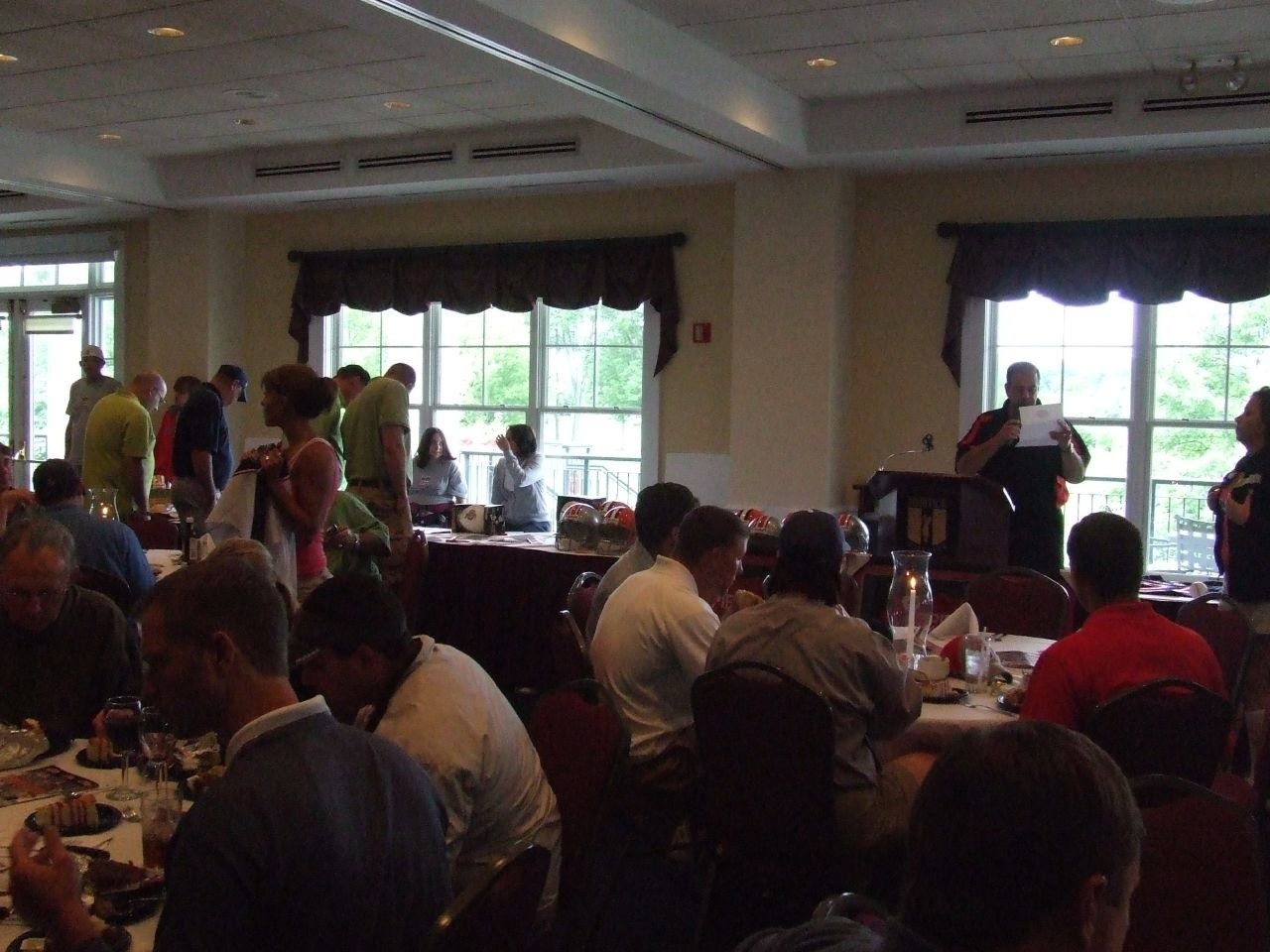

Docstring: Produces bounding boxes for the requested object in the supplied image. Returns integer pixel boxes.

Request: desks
[0,501,1226,952]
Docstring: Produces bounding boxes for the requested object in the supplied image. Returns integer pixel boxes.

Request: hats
[82,346,104,361]
[219,365,249,403]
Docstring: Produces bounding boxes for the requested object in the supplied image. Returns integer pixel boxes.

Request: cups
[963,633,999,707]
[140,787,184,879]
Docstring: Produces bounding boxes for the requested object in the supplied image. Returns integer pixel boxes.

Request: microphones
[1007,414,1022,448]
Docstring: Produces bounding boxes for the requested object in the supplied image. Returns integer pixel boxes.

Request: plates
[24,803,122,837]
[76,747,148,770]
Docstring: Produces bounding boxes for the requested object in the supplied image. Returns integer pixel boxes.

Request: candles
[102,506,109,519]
[907,576,917,659]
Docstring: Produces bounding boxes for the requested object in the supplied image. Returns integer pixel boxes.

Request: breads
[1003,688,1026,707]
[734,589,760,609]
[86,737,109,760]
[21,718,43,738]
[917,678,948,697]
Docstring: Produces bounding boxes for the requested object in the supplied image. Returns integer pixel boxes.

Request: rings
[276,452,281,457]
[265,455,270,461]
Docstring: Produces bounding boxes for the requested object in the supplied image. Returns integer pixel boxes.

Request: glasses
[0,578,69,606]
[153,388,167,405]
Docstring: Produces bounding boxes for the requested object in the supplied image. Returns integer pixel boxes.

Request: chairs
[1121,773,1270,952]
[548,608,697,952]
[526,675,630,924]
[566,569,602,633]
[1175,592,1256,714]
[692,661,841,951]
[418,844,551,952]
[965,567,1069,640]
[1089,679,1231,808]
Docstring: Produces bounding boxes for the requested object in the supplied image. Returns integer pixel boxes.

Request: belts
[350,478,395,488]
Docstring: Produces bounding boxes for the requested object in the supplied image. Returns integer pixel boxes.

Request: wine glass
[139,704,181,815]
[101,695,145,801]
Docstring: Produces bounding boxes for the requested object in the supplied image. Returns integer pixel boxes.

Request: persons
[241,363,343,609]
[93,539,297,750]
[340,364,420,636]
[705,507,940,860]
[739,717,1148,952]
[1020,511,1230,737]
[409,427,466,504]
[281,364,371,467]
[154,364,248,552]
[587,502,766,799]
[955,362,1091,579]
[491,424,551,533]
[1207,385,1270,792]
[0,343,166,743]
[584,481,701,650]
[322,491,391,581]
[8,555,450,952]
[291,572,562,952]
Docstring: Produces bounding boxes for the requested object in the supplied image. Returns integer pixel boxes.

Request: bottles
[182,516,200,563]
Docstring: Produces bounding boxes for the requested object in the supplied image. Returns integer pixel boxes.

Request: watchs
[1219,496,1231,511]
[1061,442,1074,453]
[354,532,360,548]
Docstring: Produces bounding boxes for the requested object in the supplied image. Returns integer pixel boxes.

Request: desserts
[34,792,99,827]
